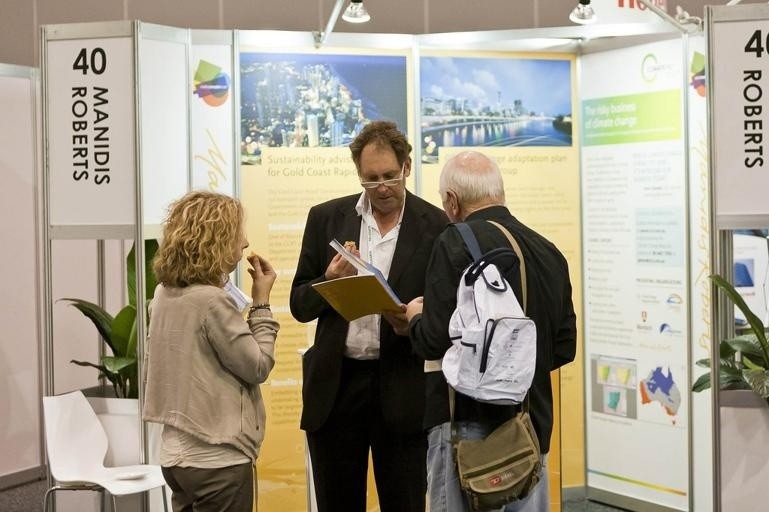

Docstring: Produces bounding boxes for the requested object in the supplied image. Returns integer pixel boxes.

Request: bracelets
[246,303,270,318]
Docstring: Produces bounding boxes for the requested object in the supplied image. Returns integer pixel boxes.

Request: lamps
[312,0,371,51]
[568,0,688,36]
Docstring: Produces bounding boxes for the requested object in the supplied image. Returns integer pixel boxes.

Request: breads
[344,241,355,248]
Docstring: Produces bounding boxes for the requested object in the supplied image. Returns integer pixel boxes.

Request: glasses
[361,160,405,189]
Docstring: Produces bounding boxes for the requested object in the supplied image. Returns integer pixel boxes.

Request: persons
[404,148,579,512]
[137,189,282,512]
[285,118,454,511]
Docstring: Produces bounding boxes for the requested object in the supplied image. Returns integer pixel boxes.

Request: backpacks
[441,222,537,406]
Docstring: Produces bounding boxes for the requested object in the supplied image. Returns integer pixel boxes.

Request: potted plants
[49,240,172,511]
[693,272,769,512]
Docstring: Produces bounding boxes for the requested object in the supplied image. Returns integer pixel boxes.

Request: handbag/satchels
[452,409,542,510]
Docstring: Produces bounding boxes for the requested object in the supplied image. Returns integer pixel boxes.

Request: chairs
[42,389,172,512]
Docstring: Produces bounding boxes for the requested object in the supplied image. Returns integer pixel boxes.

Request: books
[225,282,253,314]
[311,236,407,324]
